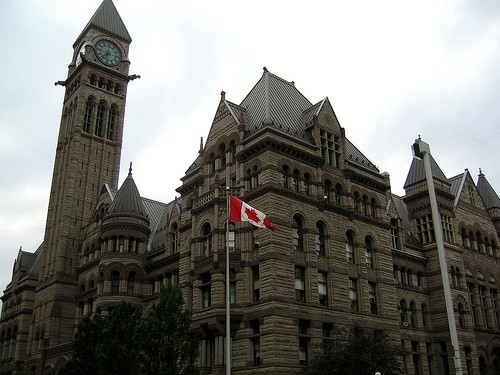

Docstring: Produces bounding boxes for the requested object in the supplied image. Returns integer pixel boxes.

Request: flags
[229,194,278,231]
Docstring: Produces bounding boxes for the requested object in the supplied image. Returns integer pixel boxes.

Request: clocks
[61,0,135,80]
